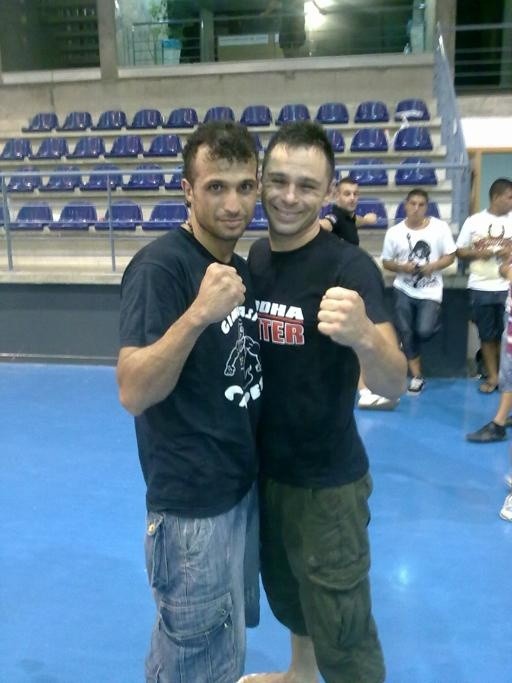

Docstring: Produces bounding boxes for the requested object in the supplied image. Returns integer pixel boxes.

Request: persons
[466,256,512,442]
[380,189,457,396]
[112,120,266,683]
[240,121,411,682]
[457,177,512,395]
[319,176,401,410]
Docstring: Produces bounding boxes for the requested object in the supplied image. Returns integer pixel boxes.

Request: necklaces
[414,218,426,228]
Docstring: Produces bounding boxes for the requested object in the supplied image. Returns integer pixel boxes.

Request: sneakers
[467,421,505,442]
[359,394,391,410]
[408,375,424,395]
[500,494,512,520]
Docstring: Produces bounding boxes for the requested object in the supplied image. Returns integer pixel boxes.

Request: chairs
[0,100,443,231]
